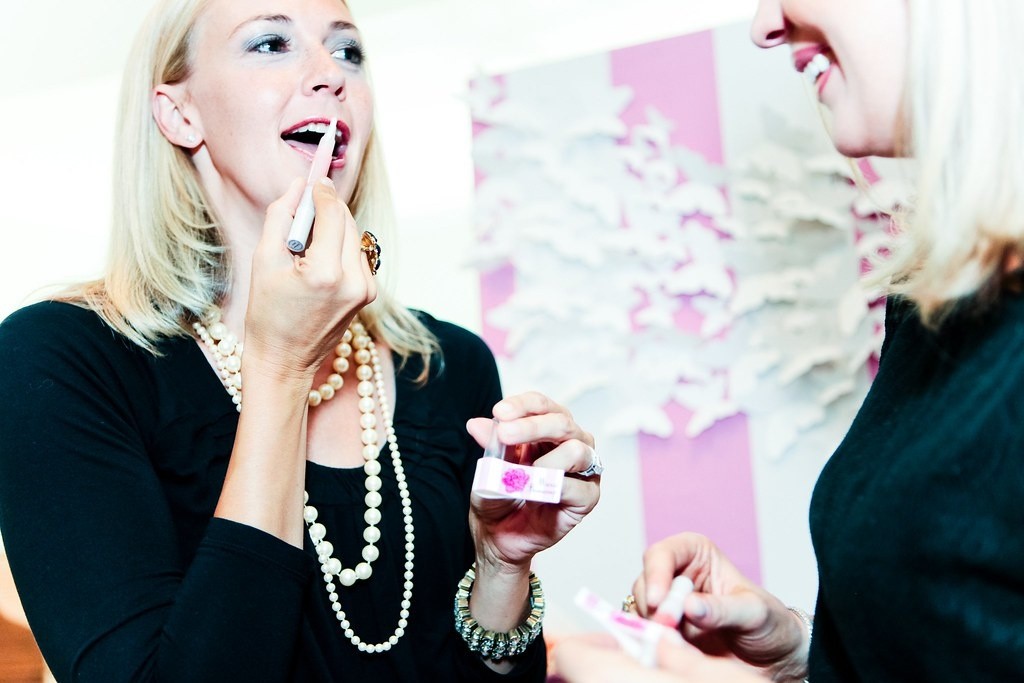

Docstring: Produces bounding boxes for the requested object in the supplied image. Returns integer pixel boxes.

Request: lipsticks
[287,118,337,252]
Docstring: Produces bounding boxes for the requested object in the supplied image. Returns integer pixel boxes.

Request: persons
[0,0,600,683]
[557,0,1024,683]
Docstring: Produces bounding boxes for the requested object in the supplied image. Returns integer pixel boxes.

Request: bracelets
[786,606,813,638]
[454,563,544,659]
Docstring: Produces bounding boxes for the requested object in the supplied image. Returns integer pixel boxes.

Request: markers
[286,113,340,253]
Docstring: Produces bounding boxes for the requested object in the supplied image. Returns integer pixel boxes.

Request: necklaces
[196,305,415,652]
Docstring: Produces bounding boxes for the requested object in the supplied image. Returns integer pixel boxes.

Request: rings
[360,230,381,274]
[578,447,604,477]
[622,596,637,612]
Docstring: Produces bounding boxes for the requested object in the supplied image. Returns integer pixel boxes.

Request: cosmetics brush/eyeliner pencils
[649,575,695,629]
